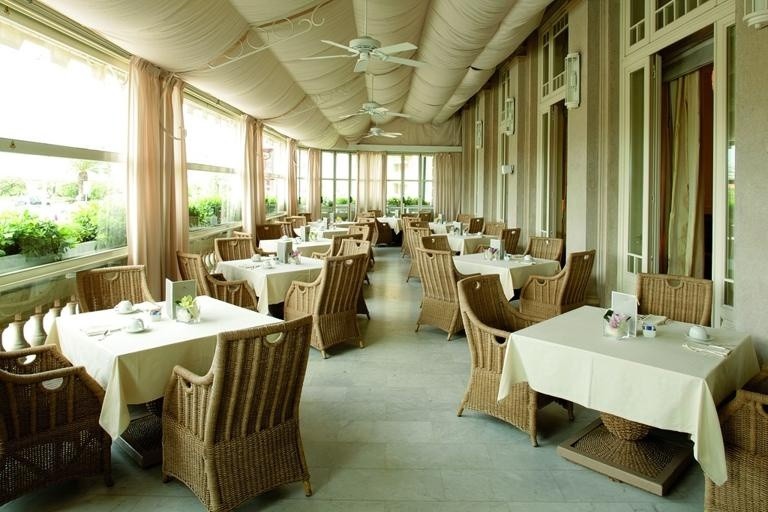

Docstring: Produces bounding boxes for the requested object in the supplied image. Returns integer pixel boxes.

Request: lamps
[564,51,582,110]
[743,0,767,31]
[475,120,483,149]
[502,98,514,134]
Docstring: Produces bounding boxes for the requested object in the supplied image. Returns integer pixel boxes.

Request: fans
[346,118,402,143]
[303,1,432,74]
[338,75,414,122]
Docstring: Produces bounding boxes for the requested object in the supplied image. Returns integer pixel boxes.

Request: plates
[117,310,135,313]
[127,329,145,333]
[686,332,714,341]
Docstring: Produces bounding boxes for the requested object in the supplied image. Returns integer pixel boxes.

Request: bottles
[150,311,161,321]
[642,325,656,337]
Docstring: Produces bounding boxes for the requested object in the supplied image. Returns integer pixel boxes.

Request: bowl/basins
[119,301,132,311]
[525,255,532,260]
[252,254,260,261]
[129,318,144,330]
[689,325,707,339]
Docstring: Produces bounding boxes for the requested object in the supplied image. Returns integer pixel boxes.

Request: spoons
[98,329,111,341]
